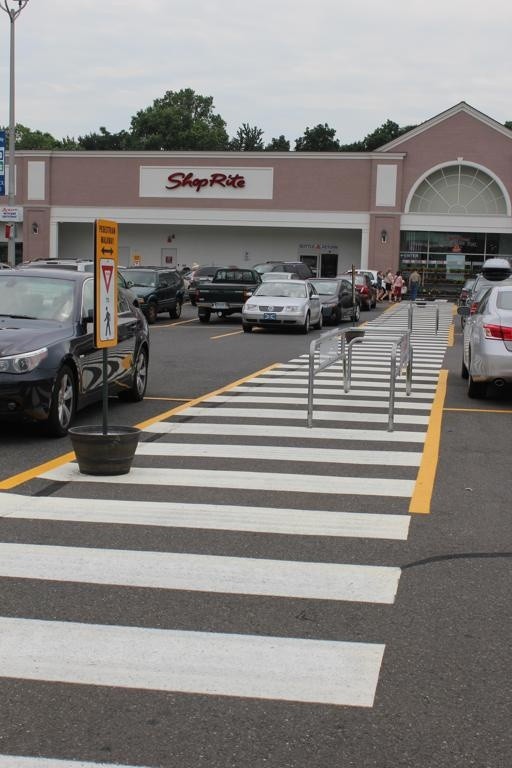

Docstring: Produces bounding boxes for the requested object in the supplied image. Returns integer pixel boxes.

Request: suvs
[119,265,184,323]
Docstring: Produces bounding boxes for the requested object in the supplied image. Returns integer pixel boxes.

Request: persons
[379,268,394,302]
[393,270,404,302]
[375,269,384,303]
[190,260,200,283]
[408,268,421,301]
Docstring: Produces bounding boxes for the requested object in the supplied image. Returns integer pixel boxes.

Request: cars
[457,258,512,399]
[0,263,149,437]
[177,262,384,334]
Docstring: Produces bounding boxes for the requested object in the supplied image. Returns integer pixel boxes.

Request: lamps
[381,229,387,244]
[32,222,39,235]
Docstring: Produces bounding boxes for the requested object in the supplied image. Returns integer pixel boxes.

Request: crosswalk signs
[94,220,119,349]
